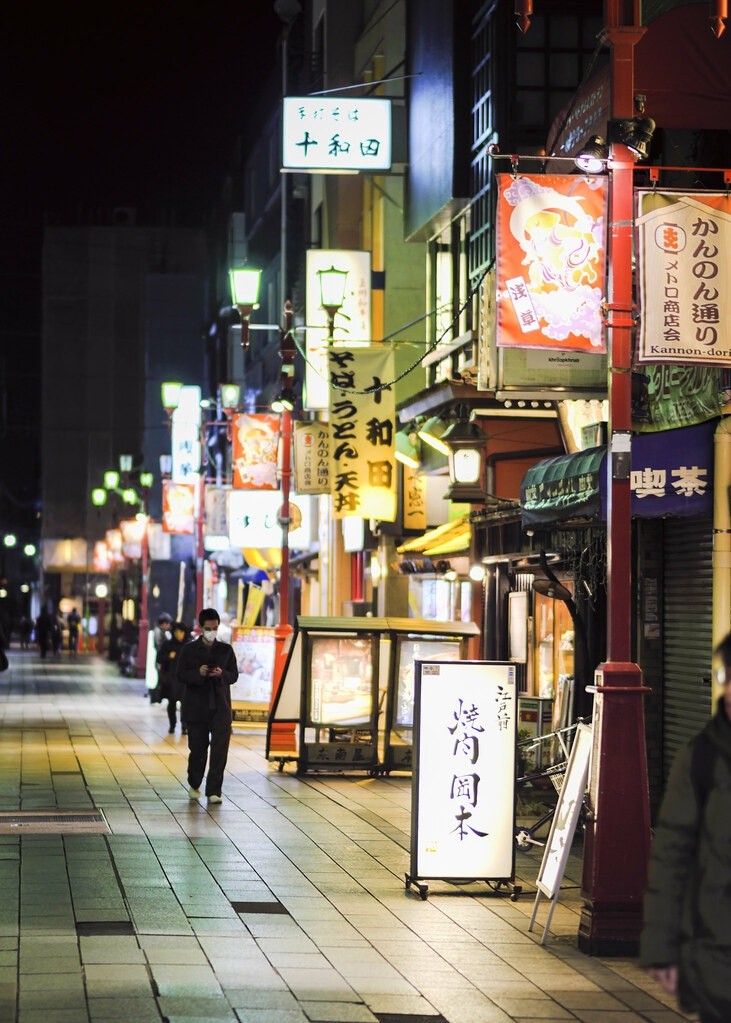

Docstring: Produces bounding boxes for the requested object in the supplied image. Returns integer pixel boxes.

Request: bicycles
[440,714,594,886]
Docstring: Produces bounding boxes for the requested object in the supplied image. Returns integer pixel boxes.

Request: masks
[202,628,218,641]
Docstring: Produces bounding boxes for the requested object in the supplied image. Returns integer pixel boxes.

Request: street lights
[86,378,242,701]
[228,254,348,750]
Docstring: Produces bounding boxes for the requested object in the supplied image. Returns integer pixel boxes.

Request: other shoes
[169,727,174,732]
[182,729,188,734]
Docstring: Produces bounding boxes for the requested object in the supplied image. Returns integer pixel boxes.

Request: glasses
[715,666,731,685]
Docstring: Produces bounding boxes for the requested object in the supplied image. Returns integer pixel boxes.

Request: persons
[149,613,178,708]
[176,607,240,804]
[215,614,233,645]
[155,623,193,737]
[643,634,731,1023]
[18,605,85,658]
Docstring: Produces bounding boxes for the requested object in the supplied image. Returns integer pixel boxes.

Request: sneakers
[208,795,221,804]
[188,786,201,800]
